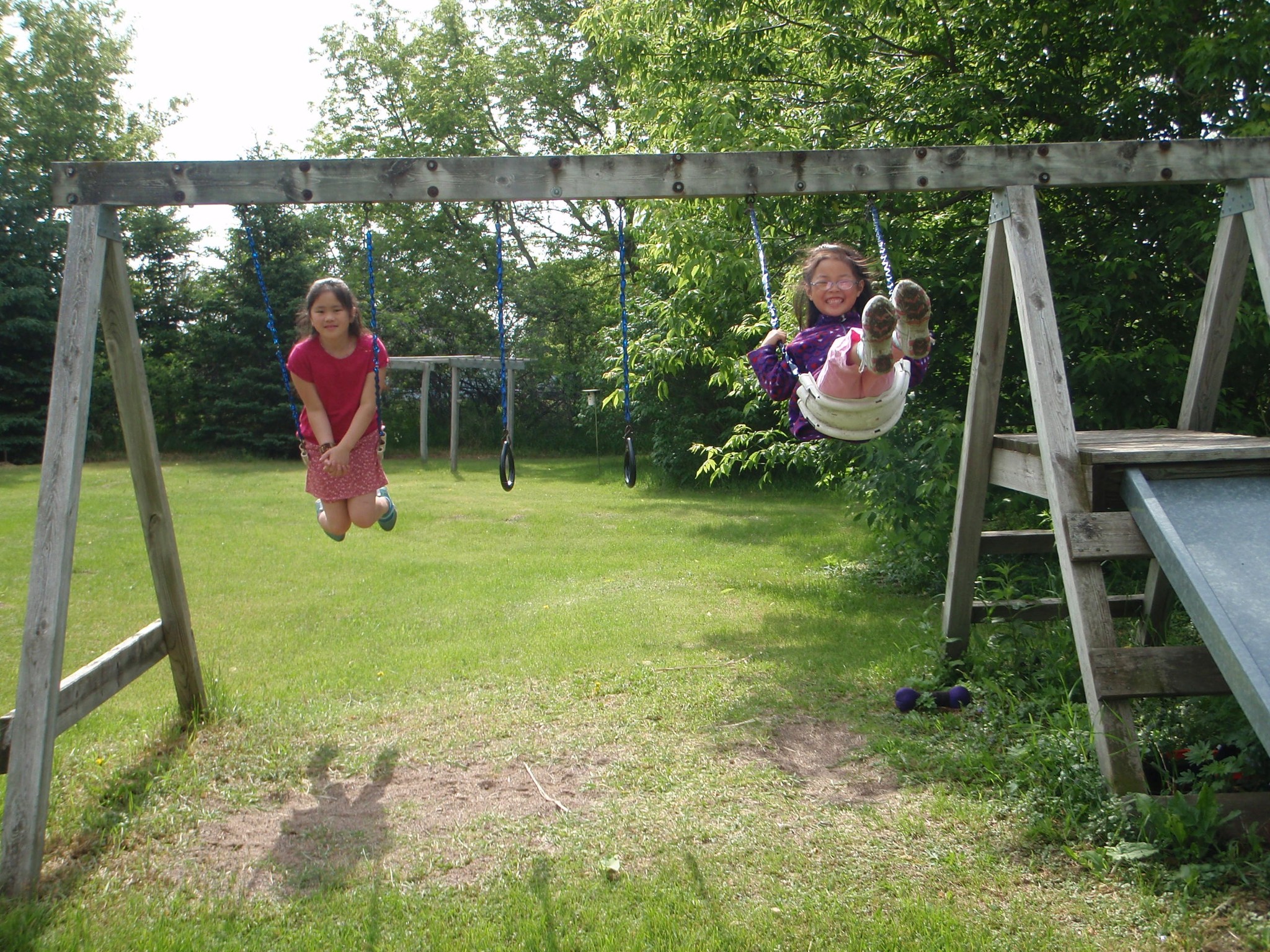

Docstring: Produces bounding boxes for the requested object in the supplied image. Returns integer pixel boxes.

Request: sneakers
[859,294,898,375]
[890,279,931,359]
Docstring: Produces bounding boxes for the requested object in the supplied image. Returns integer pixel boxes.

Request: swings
[744,190,912,441]
[236,201,389,469]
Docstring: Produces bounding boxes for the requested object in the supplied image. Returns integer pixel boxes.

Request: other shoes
[315,497,346,542]
[374,486,398,531]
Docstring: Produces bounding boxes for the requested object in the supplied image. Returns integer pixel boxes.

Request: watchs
[318,442,338,455]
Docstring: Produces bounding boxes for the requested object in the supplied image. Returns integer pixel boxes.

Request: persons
[287,275,397,540]
[746,243,930,444]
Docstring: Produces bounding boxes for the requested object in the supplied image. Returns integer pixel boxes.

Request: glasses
[809,280,859,291]
